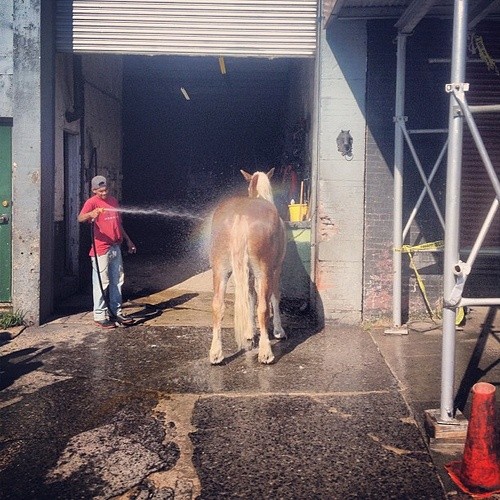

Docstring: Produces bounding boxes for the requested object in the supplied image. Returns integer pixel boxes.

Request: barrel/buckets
[288,204,307,222]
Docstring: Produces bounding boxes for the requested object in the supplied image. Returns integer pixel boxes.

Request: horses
[208,167,287,366]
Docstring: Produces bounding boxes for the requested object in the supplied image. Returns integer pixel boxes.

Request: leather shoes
[95,318,116,328]
[111,314,133,324]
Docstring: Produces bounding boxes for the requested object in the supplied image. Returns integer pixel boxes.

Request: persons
[78,175,136,329]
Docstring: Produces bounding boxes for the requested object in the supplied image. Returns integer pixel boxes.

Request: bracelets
[88,212,92,219]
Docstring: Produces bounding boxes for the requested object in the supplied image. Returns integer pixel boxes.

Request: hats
[91,175,107,190]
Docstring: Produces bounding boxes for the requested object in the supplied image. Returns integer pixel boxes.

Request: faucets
[96,207,105,213]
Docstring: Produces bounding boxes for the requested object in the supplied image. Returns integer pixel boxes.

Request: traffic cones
[443,382,500,495]
